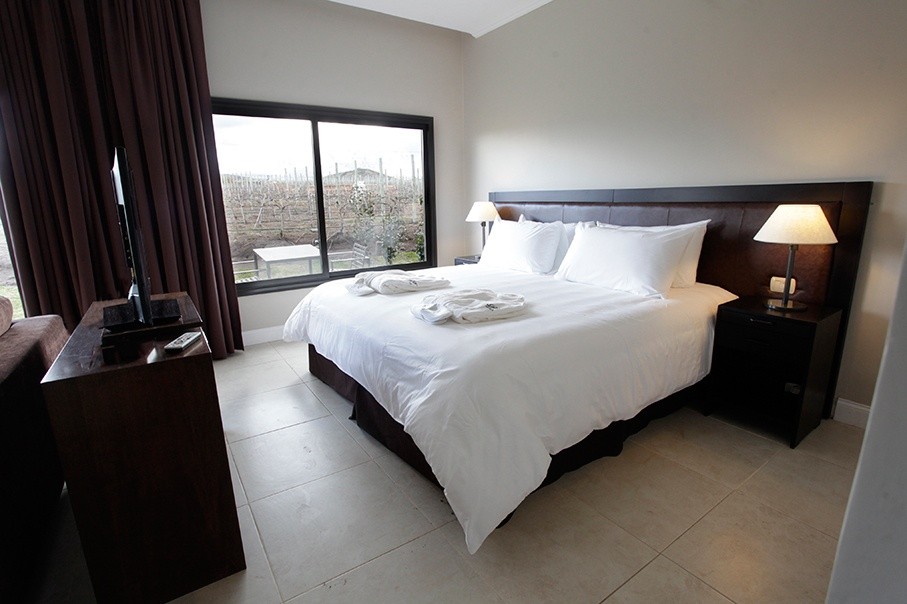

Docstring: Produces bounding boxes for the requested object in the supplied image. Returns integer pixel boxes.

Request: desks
[38,291,248,604]
[252,244,320,279]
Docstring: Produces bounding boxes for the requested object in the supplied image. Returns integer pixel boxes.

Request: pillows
[474,212,712,301]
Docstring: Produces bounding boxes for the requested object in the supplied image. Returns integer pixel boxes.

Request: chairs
[328,242,370,272]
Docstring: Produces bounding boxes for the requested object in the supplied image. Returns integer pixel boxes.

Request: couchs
[0,295,71,604]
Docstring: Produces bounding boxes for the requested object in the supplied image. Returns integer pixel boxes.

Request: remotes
[164,331,201,354]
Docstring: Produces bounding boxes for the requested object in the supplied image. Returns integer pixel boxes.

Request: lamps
[751,204,839,315]
[465,201,502,262]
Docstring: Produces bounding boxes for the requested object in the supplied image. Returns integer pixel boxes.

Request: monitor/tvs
[98,145,182,331]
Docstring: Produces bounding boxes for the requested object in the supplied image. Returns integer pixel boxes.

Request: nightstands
[707,290,853,450]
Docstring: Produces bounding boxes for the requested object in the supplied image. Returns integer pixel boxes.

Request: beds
[278,180,875,550]
